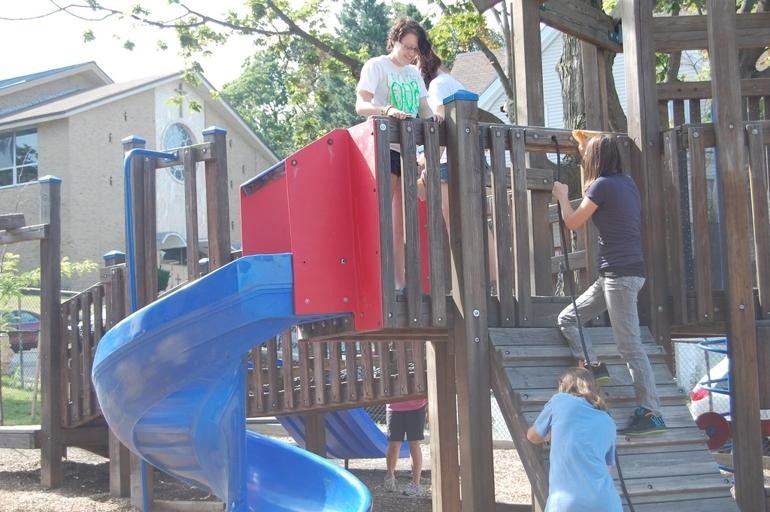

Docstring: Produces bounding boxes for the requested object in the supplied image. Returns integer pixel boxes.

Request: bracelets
[384,104,394,116]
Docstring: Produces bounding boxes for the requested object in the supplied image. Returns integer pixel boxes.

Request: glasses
[398,39,422,55]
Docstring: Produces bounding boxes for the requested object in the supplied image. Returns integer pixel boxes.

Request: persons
[552,129,667,438]
[526,366,623,512]
[411,46,500,299]
[353,19,445,305]
[385,398,428,498]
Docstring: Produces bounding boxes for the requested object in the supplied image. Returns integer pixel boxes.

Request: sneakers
[583,361,610,385]
[623,405,668,438]
[402,480,422,497]
[383,472,397,492]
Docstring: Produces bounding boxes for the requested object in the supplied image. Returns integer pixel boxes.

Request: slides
[91,253,378,511]
[246,360,410,469]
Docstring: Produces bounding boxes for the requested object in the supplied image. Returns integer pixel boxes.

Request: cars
[0,309,81,353]
[687,354,731,424]
